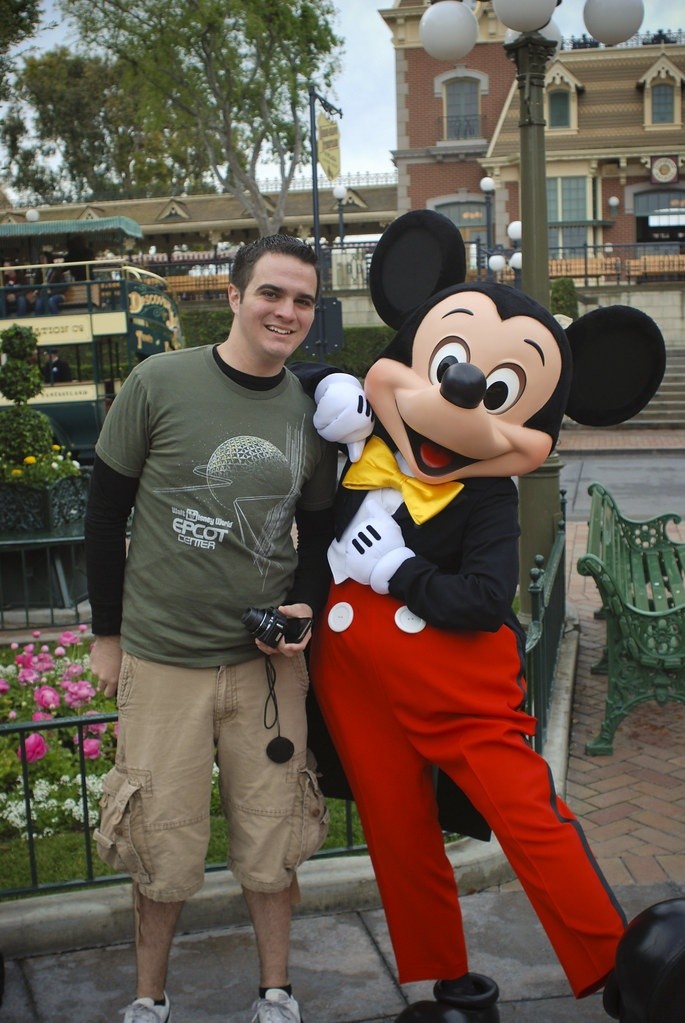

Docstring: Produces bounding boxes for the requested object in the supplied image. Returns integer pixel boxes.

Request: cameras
[240,606,290,648]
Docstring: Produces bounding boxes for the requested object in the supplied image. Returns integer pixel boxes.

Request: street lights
[332,184,347,254]
[479,175,522,291]
[420,0,644,646]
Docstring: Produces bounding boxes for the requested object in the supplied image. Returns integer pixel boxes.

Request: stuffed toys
[288,209,685,1023]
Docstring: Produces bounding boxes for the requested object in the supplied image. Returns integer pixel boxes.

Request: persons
[85,233,338,1023]
[31,251,68,317]
[0,260,28,317]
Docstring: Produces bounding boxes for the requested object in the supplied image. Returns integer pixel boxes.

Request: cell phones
[282,617,313,644]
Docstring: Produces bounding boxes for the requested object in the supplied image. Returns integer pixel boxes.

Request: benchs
[577,482,685,757]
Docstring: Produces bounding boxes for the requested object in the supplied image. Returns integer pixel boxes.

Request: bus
[0,217,186,456]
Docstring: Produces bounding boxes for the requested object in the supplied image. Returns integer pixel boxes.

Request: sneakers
[117,989,170,1023]
[250,988,301,1023]
